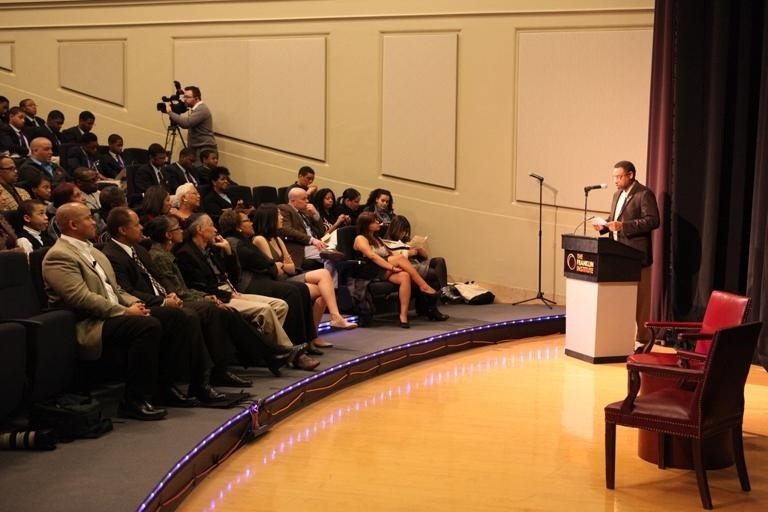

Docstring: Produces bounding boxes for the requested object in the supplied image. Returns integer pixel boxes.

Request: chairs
[605,289,764,510]
[0,139,414,441]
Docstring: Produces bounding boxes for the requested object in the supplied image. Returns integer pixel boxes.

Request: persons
[593,160,661,351]
[44,134,200,420]
[103,209,334,401]
[165,88,218,167]
[1,95,96,265]
[336,188,450,329]
[203,150,357,345]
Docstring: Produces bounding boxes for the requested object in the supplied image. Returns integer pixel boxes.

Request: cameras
[343,215,349,221]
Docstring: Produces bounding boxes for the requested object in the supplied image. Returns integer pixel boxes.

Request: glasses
[0,166,18,172]
[464,281,474,284]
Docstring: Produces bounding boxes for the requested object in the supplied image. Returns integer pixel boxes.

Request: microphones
[563,216,596,236]
[584,184,607,193]
[528,171,543,181]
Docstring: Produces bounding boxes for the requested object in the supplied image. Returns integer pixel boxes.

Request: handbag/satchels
[383,238,410,260]
[454,282,495,304]
[30,392,113,440]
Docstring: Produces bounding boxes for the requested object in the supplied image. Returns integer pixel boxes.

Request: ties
[131,246,167,298]
[116,153,124,169]
[186,169,194,186]
[158,171,163,184]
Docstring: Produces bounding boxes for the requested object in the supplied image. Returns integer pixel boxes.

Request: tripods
[163,125,187,167]
[512,180,557,309]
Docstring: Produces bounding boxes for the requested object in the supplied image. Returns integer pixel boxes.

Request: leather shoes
[439,286,465,305]
[264,340,334,376]
[117,368,252,421]
[398,314,410,328]
[426,307,450,321]
[330,320,358,330]
[19,131,29,156]
